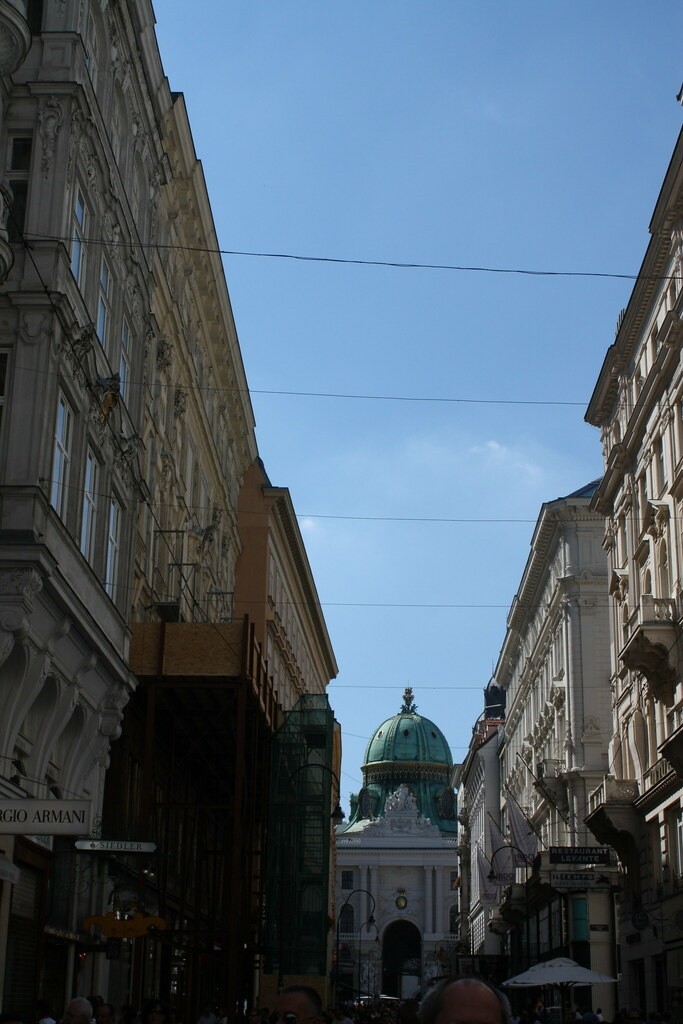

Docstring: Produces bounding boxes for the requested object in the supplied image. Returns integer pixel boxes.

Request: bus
[354,995,400,1007]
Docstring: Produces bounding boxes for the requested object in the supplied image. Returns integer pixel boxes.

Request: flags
[477,847,500,906]
[507,791,538,868]
[489,816,515,885]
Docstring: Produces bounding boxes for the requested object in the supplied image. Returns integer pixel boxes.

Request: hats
[383,1008,392,1016]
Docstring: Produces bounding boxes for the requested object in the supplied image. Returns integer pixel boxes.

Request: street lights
[482,844,530,969]
[334,889,379,1006]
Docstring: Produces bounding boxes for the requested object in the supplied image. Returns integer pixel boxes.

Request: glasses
[269,1012,316,1024]
[628,1017,642,1021]
[382,1015,388,1017]
[149,1010,162,1015]
[246,1014,257,1017]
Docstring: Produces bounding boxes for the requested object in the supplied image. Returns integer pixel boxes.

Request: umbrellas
[503,957,619,1024]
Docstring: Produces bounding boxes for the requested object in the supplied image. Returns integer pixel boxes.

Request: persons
[0,993,683,1024]
[416,977,511,1024]
[276,986,322,1024]
